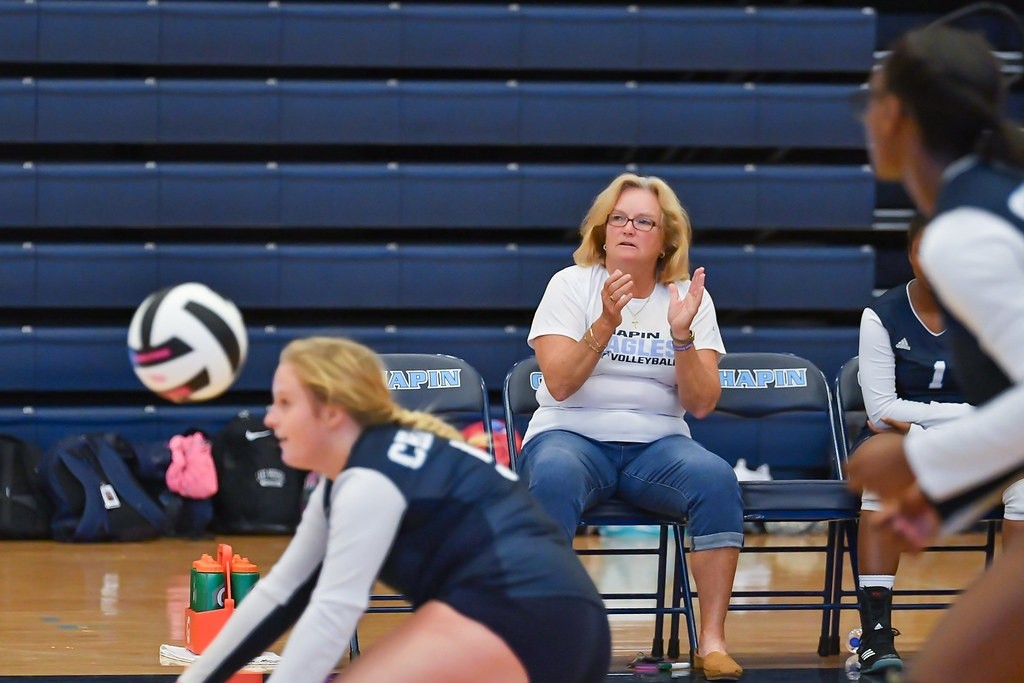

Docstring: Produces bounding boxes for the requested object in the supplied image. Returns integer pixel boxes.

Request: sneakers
[856,628,904,673]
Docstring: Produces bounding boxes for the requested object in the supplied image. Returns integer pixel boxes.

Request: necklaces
[622,280,657,329]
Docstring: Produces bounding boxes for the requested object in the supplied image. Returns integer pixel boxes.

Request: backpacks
[47,431,170,541]
[212,417,298,535]
[1,433,44,539]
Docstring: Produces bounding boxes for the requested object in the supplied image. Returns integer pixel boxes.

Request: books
[158,645,281,675]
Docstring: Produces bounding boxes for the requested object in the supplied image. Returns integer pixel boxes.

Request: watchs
[670,328,695,344]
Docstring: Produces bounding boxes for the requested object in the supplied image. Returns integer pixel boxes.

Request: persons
[512,173,745,682]
[174,336,610,683]
[844,27,1024,683]
[849,211,1024,673]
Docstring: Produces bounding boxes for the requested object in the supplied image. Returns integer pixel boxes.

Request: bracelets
[589,324,609,350]
[671,342,695,352]
[582,334,603,353]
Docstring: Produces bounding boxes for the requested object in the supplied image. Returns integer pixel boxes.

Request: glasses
[607,213,663,232]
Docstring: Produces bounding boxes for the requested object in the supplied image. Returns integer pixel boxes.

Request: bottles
[845,629,863,654]
[190,554,225,611]
[233,552,260,608]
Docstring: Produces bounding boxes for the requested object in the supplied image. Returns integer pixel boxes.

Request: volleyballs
[126,281,249,404]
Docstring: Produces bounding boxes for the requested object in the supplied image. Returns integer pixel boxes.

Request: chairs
[351,352,496,661]
[667,353,863,666]
[828,355,995,655]
[502,356,697,668]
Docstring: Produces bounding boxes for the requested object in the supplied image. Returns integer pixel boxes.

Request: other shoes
[693,649,742,681]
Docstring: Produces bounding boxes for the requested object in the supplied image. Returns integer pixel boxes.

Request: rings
[608,296,618,303]
